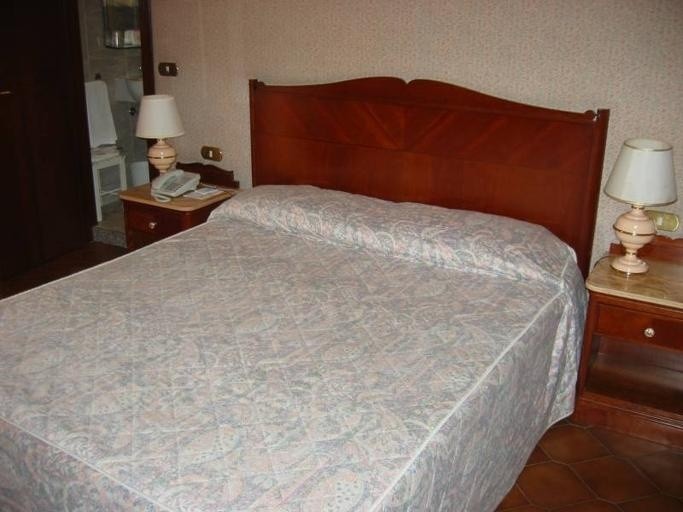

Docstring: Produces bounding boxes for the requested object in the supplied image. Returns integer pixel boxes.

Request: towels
[85,79,118,148]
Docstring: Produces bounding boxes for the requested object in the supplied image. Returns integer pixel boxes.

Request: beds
[0,77,611,512]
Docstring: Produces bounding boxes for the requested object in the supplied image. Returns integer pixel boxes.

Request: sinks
[116,75,143,102]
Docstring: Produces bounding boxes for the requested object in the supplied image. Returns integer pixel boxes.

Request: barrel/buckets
[130,161,150,186]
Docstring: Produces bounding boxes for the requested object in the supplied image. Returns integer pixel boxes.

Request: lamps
[603,136,678,279]
[134,93,186,176]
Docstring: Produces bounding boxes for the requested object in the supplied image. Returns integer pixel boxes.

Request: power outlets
[643,210,680,233]
[200,145,223,162]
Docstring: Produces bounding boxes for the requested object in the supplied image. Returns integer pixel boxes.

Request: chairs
[84,80,128,222]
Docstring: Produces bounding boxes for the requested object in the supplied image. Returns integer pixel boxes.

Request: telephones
[151,169,201,197]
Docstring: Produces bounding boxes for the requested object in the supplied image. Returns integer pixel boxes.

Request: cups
[109,31,139,46]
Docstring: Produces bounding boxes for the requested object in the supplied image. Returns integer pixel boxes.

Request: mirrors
[101,0,142,51]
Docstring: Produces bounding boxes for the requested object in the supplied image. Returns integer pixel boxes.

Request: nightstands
[568,235,683,450]
[117,162,241,254]
